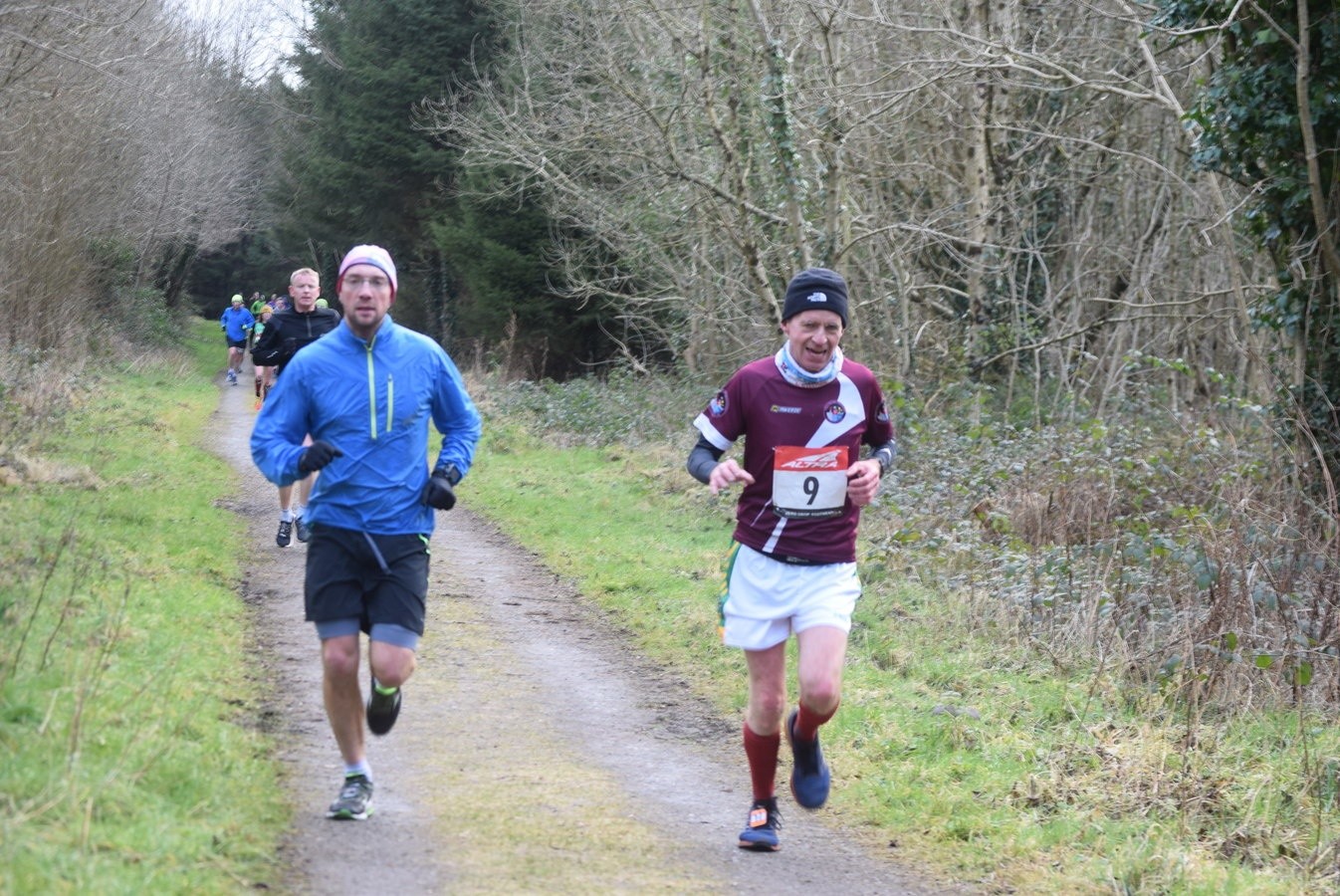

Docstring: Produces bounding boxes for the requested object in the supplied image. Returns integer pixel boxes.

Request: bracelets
[868,457,887,480]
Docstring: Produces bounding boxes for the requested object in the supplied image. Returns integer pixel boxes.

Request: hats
[316,299,328,310]
[782,267,848,330]
[260,305,272,314]
[337,244,399,305]
[231,295,243,302]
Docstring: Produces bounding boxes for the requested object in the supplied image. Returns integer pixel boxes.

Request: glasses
[342,275,389,292]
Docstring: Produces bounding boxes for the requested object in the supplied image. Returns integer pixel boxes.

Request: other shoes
[256,397,262,409]
[227,369,237,385]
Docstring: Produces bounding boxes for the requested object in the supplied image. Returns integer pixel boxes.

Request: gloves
[241,325,247,330]
[222,326,226,331]
[421,470,457,511]
[298,439,342,473]
[277,336,297,360]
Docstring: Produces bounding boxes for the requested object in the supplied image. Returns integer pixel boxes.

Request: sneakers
[366,676,401,735]
[784,711,830,809]
[740,797,784,851]
[324,771,374,820]
[294,515,312,543]
[277,514,294,547]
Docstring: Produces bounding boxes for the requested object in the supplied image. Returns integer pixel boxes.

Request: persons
[314,297,329,310]
[250,266,341,549]
[220,290,292,410]
[249,244,483,822]
[686,268,892,853]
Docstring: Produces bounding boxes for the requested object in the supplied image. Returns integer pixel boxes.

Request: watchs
[431,462,463,486]
